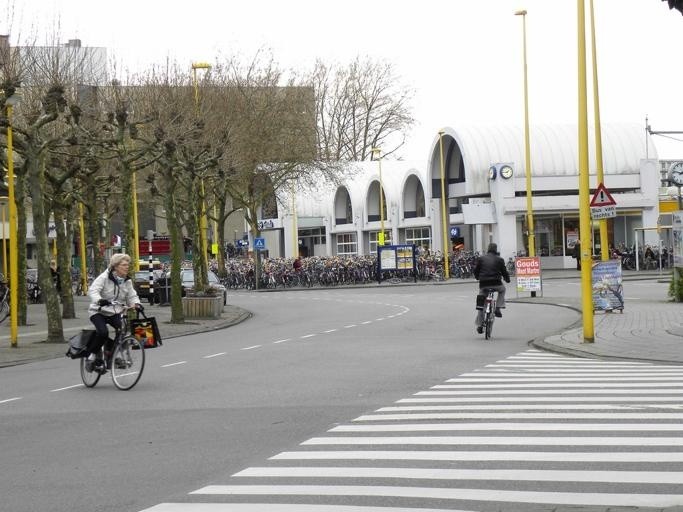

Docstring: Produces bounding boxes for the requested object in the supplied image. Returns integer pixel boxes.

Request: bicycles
[472,274,509,342]
[72,297,153,388]
[0,275,41,327]
[73,273,93,297]
[502,239,674,276]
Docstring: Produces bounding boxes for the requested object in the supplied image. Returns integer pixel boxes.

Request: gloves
[135,304,144,312]
[97,297,111,307]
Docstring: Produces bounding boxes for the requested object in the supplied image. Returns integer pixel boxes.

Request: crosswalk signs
[253,238,266,250]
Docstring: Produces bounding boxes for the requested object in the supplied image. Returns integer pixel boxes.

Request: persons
[473,243,510,334]
[572,239,580,271]
[83,253,141,371]
[415,245,422,255]
[422,244,430,259]
[293,255,302,272]
[48,258,55,273]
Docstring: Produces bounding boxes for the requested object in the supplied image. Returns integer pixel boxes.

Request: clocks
[487,166,497,180]
[498,164,514,179]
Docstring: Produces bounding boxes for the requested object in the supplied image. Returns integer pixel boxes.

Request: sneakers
[495,310,502,318]
[83,355,94,372]
[476,326,484,334]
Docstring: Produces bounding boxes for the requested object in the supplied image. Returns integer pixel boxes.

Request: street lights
[370,146,387,249]
[434,127,451,281]
[513,9,539,296]
[188,51,215,273]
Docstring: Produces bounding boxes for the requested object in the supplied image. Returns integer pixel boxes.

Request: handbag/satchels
[129,306,163,350]
[65,328,98,359]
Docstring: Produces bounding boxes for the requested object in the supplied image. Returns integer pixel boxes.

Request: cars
[124,256,164,304]
[23,264,81,297]
[161,268,229,307]
